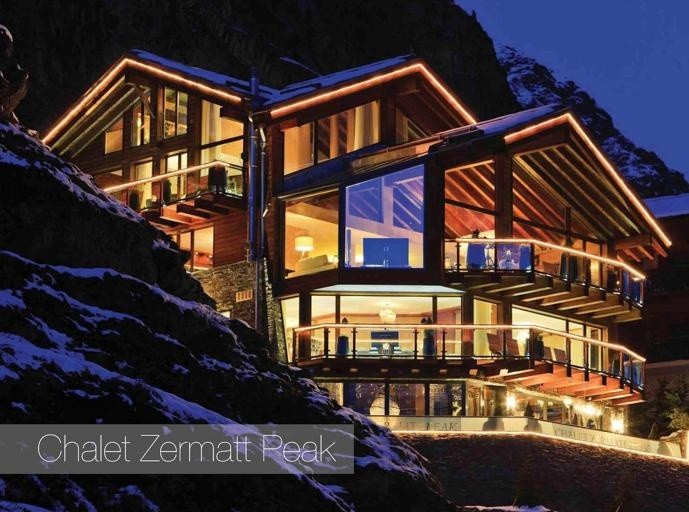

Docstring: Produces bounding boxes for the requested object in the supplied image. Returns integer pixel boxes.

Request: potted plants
[667,407,689,459]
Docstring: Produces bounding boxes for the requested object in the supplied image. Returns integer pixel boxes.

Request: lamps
[380,306,397,324]
[295,235,315,257]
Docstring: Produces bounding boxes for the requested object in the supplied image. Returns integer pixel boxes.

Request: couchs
[288,255,336,278]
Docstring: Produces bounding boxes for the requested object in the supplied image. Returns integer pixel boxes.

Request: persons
[606,264,617,291]
[537,335,544,359]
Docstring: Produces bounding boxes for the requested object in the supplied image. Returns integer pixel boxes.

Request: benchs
[488,333,525,360]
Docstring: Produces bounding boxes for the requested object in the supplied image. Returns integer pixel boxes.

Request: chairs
[543,346,552,361]
[554,348,566,363]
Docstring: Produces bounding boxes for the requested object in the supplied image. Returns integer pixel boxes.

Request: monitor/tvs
[363,237,409,265]
[370,331,402,351]
[495,244,534,271]
